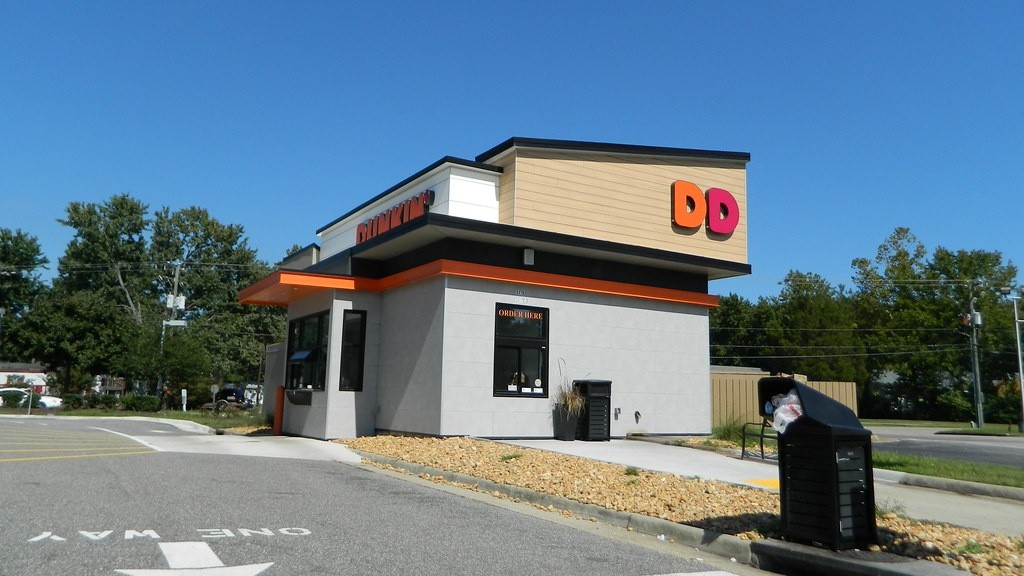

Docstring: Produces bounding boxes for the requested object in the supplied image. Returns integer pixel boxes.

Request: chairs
[741,418,775,459]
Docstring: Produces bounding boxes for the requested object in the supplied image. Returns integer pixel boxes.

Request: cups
[299,383,303,388]
[307,385,312,388]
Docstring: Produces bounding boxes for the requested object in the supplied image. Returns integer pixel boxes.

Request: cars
[0,387,64,410]
[214,382,265,414]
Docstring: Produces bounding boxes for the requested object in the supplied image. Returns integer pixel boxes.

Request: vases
[552,406,578,441]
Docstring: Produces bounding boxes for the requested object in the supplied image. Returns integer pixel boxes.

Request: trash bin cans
[757,375,878,552]
[571,379,612,442]
